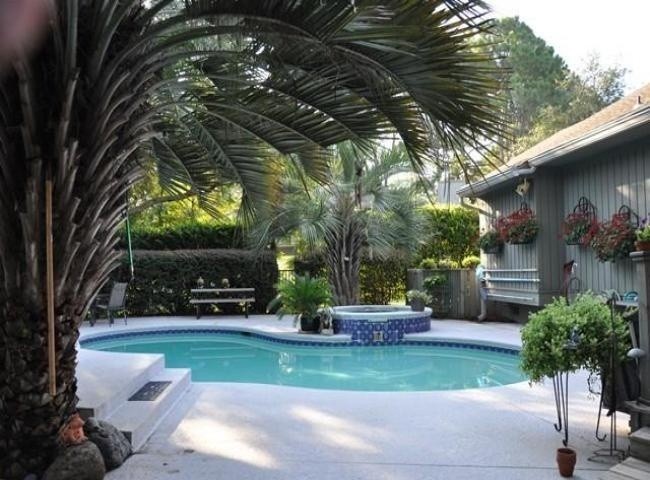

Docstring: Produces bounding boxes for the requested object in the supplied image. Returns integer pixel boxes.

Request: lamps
[513,180,530,202]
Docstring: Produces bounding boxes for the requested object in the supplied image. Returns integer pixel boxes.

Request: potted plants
[462,254,480,270]
[272,271,336,332]
[404,288,433,311]
[634,225,650,252]
[422,257,434,269]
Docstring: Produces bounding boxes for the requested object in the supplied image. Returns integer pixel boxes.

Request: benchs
[189,276,256,320]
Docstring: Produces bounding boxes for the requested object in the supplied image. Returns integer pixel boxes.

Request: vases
[556,449,577,478]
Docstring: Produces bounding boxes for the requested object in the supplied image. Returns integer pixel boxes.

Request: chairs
[90,282,128,327]
